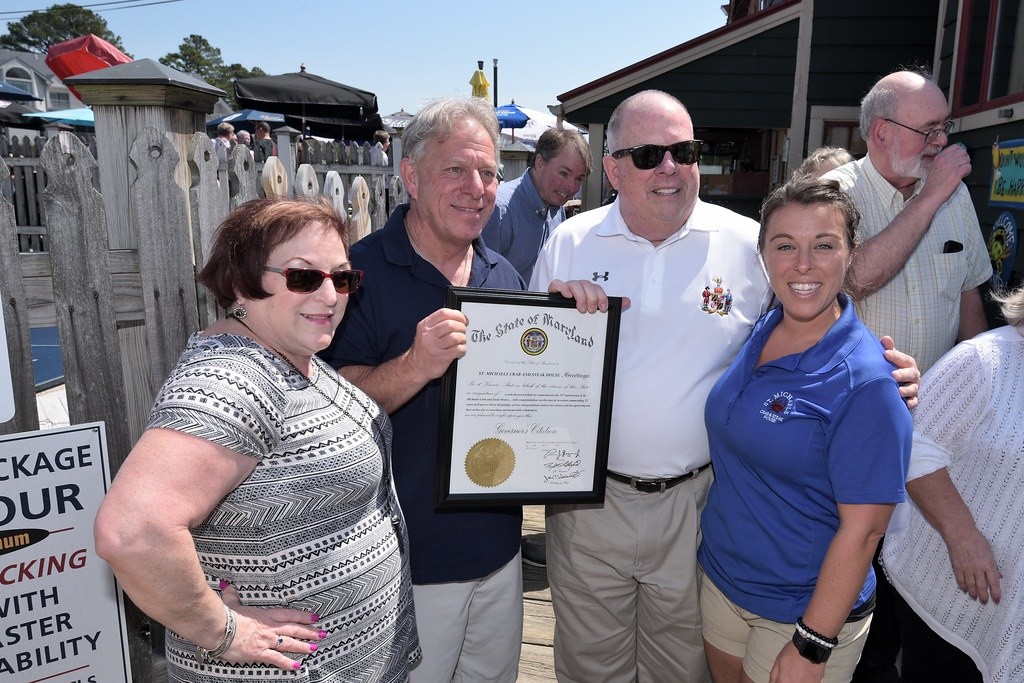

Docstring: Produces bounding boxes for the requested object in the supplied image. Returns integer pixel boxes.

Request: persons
[851,294,1024,683]
[697,178,912,683]
[372,129,390,214]
[528,90,920,683]
[94,198,423,683]
[317,97,529,683]
[819,72,993,374]
[212,124,302,184]
[482,129,592,284]
[801,147,854,178]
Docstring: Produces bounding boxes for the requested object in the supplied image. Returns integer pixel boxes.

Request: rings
[276,635,283,649]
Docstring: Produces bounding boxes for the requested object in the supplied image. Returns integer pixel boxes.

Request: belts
[607,463,712,494]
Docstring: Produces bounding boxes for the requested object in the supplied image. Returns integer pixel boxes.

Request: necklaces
[232,315,405,554]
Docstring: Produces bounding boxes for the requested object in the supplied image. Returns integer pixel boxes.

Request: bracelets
[796,616,838,648]
[195,605,236,664]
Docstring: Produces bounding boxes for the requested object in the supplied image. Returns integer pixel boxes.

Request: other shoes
[522,541,547,567]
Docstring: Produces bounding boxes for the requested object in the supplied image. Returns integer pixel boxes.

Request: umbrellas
[495,99,578,143]
[470,70,491,100]
[205,62,384,140]
[0,80,94,126]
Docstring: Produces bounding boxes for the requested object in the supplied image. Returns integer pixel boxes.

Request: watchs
[792,630,831,663]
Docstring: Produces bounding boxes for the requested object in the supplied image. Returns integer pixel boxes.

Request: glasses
[883,118,955,144]
[262,266,363,294]
[612,139,704,170]
